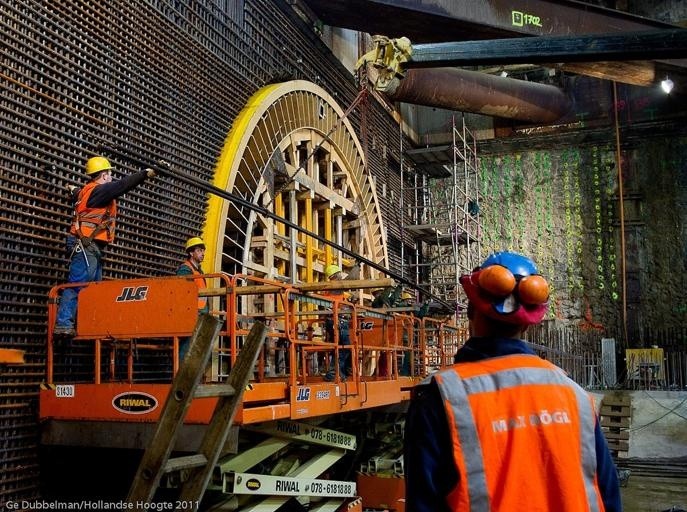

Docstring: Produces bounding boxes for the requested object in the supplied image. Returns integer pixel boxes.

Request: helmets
[327,265,341,277]
[85,156,115,175]
[402,293,413,299]
[461,253,549,325]
[185,238,206,252]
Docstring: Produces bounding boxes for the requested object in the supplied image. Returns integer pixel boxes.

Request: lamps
[660,74,675,94]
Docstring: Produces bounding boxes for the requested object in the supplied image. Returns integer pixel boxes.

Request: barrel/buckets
[616,467,630,488]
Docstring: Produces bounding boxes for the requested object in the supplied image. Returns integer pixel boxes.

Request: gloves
[66,184,79,194]
[146,159,170,177]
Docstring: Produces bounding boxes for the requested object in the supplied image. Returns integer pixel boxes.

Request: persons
[52,155,171,339]
[175,235,210,382]
[372,283,403,374]
[402,250,623,511]
[401,292,433,377]
[323,263,360,385]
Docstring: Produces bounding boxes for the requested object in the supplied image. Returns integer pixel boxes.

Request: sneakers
[55,327,74,334]
